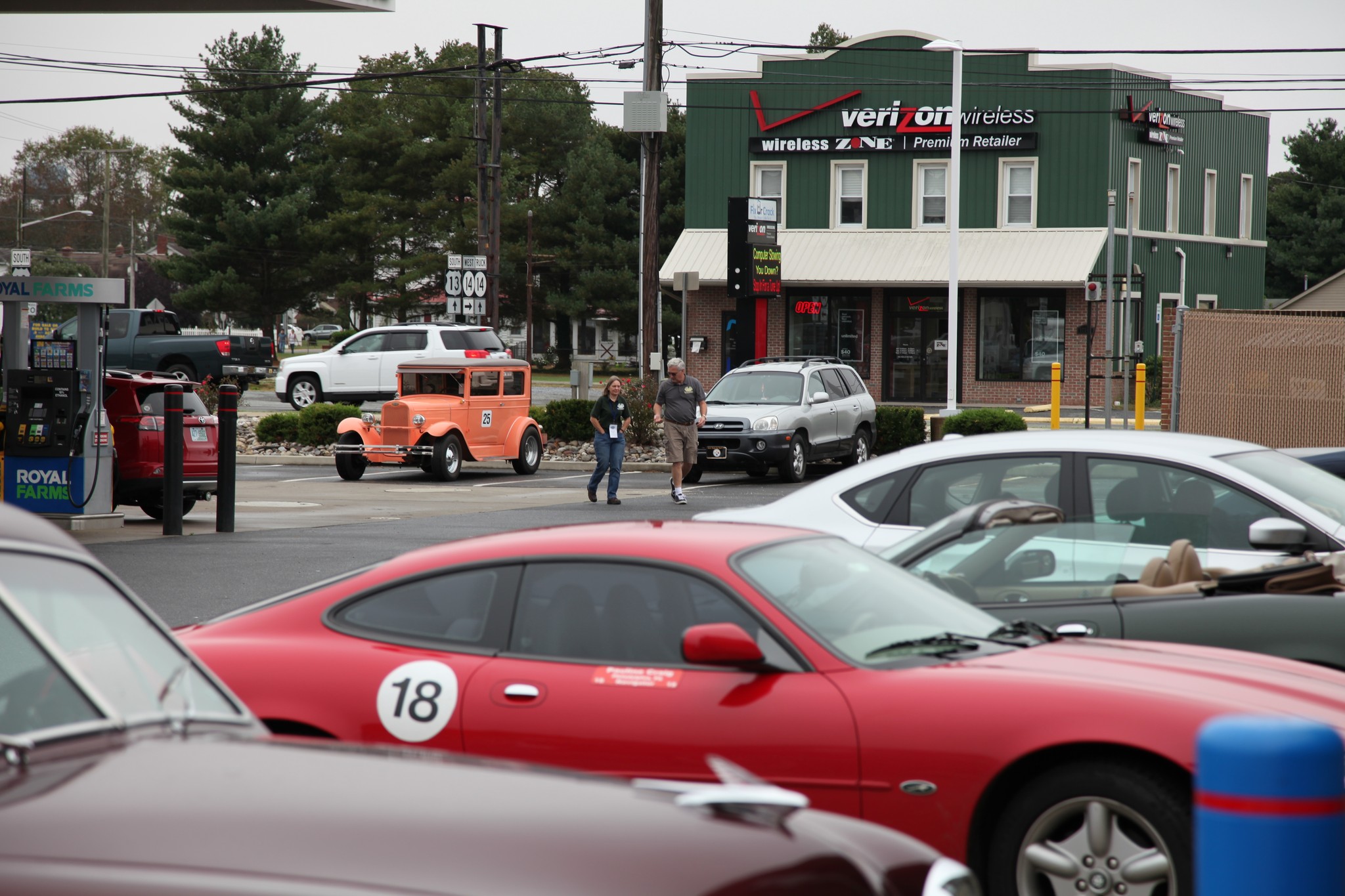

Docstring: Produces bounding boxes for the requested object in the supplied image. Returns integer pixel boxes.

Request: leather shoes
[607,497,621,504]
[587,484,598,502]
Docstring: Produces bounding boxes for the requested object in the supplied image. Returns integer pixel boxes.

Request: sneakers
[674,493,687,504]
[670,477,676,501]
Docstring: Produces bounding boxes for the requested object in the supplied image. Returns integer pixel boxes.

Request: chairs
[742,379,768,402]
[344,576,686,663]
[978,470,1234,549]
[413,376,517,395]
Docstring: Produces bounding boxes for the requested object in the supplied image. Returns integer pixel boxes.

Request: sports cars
[859,502,1344,671]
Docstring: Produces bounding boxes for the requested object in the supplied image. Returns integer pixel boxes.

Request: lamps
[1226,246,1233,257]
[1150,240,1157,252]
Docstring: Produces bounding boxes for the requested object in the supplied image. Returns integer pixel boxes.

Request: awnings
[658,229,1112,290]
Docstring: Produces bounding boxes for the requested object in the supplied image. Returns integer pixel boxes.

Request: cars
[273,323,305,347]
[334,354,543,482]
[1175,445,1345,512]
[1027,316,1065,381]
[3,519,1345,891]
[691,443,1345,604]
[0,506,985,896]
[891,328,948,360]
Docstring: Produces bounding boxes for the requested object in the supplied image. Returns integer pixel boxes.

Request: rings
[702,425,703,426]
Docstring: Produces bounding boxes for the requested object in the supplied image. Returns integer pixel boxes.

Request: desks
[892,361,948,400]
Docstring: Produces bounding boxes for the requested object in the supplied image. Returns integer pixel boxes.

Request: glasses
[666,369,681,377]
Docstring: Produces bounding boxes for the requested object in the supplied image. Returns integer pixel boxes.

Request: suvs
[273,321,518,411]
[301,324,346,344]
[684,356,880,482]
[102,368,218,522]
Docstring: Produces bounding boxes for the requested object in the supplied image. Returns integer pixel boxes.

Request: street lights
[14,190,94,252]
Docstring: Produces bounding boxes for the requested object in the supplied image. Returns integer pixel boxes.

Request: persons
[652,358,707,505]
[278,329,299,354]
[587,376,632,505]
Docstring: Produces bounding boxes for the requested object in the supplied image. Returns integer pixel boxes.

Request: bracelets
[702,414,707,420]
[620,429,624,433]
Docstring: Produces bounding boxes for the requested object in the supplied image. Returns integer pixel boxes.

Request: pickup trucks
[41,306,275,402]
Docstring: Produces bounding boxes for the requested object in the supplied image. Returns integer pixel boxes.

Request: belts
[664,417,695,426]
